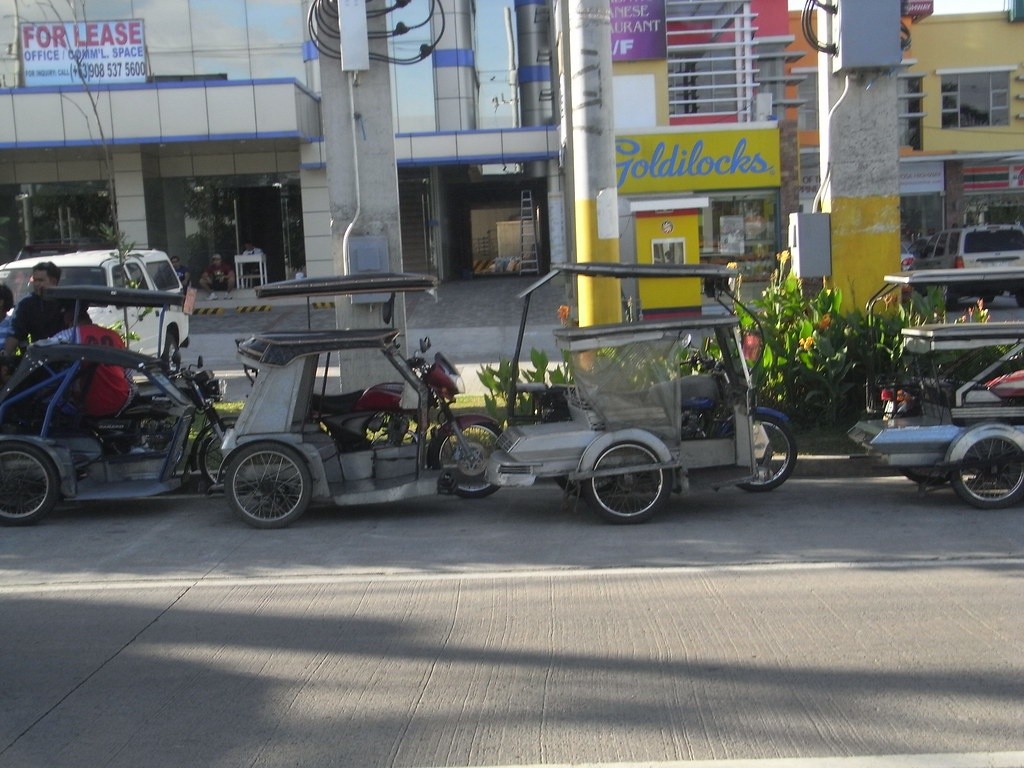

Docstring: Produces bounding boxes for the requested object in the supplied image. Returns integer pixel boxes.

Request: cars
[901,244,914,270]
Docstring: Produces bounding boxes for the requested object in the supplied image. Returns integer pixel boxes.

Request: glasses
[172,260,179,263]
[212,258,220,260]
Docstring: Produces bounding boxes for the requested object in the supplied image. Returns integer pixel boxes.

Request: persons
[25,304,145,457]
[241,240,264,272]
[0,283,19,386]
[170,255,192,294]
[12,261,75,355]
[199,253,237,302]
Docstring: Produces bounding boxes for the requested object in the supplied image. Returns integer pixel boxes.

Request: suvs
[924,224,1024,305]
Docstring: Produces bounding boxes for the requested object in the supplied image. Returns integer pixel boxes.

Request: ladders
[518,190,541,277]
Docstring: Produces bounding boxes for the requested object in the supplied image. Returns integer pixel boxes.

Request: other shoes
[224,293,233,300]
[206,292,218,300]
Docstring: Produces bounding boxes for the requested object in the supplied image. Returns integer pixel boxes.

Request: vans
[2,251,191,375]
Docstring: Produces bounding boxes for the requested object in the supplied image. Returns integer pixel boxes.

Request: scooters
[848,269,1024,511]
[0,283,243,523]
[215,271,506,528]
[482,261,800,523]
